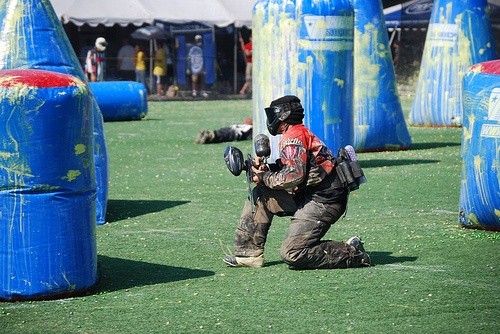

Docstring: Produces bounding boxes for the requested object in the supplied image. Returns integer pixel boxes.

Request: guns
[253,133,272,171]
[224,144,257,215]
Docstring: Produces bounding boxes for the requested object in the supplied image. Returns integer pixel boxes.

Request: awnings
[50,0,258,29]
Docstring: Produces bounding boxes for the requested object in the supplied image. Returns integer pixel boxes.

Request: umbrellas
[382,0,500,31]
[131,26,171,95]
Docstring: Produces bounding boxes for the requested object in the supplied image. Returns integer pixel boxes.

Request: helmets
[95,37,107,51]
[265,96,304,136]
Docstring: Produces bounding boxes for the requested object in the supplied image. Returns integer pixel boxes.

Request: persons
[117,37,135,81]
[131,45,147,86]
[239,36,252,95]
[223,95,372,268]
[196,119,253,145]
[85,37,108,82]
[152,40,167,96]
[187,34,209,98]
[393,41,411,74]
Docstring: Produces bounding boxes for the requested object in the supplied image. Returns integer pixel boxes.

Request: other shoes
[192,90,197,97]
[200,92,209,97]
[195,129,210,145]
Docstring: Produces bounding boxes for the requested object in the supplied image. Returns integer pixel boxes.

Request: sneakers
[347,237,371,267]
[222,254,265,269]
